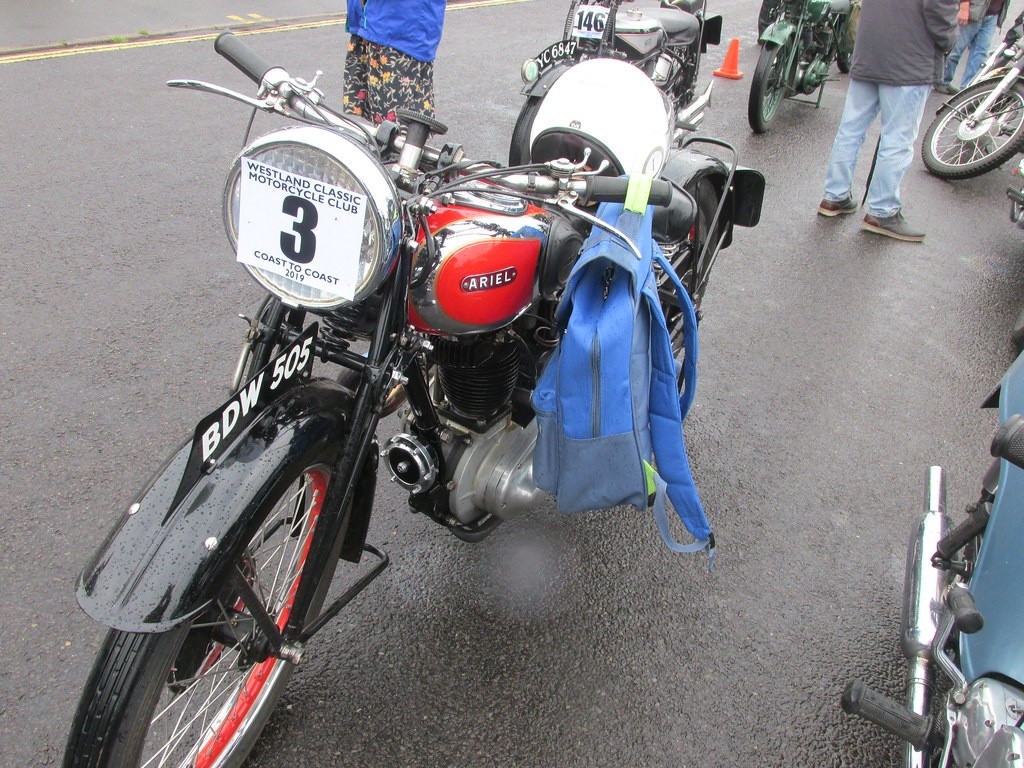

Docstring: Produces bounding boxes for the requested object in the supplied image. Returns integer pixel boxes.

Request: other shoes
[935,84,960,95]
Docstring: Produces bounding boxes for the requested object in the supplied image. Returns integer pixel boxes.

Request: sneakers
[818,190,858,217]
[861,207,925,241]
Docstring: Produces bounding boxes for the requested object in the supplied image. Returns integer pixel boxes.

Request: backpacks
[530,174,717,573]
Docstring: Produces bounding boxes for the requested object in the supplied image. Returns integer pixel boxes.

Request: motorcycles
[57,28,771,768]
[506,1,724,175]
[748,0,863,134]
[839,300,1024,768]
[920,9,1024,181]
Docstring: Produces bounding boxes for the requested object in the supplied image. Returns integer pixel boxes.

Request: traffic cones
[712,38,744,80]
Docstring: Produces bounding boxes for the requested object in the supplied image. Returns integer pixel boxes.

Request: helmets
[530,57,670,179]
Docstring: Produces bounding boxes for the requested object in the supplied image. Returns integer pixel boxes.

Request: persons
[938,0,1010,93]
[817,0,961,241]
[343,0,446,137]
